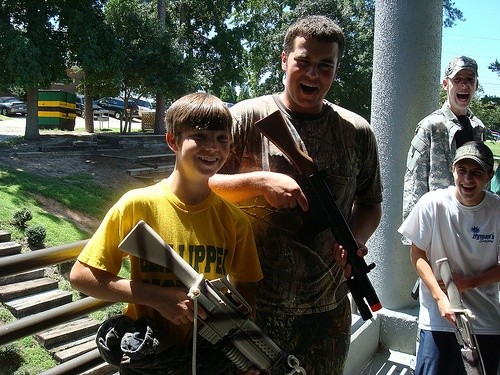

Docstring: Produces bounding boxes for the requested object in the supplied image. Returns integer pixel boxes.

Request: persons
[205,15,382,375]
[69,92,258,375]
[410,140,500,375]
[403,56,487,245]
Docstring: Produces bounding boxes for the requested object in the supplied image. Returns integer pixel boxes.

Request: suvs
[94,97,140,120]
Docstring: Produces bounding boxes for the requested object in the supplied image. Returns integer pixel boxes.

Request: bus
[132,96,172,119]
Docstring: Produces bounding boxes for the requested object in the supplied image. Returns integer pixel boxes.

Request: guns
[433,255,487,375]
[116,220,309,375]
[250,107,382,320]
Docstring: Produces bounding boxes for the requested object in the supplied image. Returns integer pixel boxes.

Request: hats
[452,141,494,171]
[444,56,479,79]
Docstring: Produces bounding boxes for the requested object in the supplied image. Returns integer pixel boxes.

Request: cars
[0,97,28,116]
[76,96,102,120]
